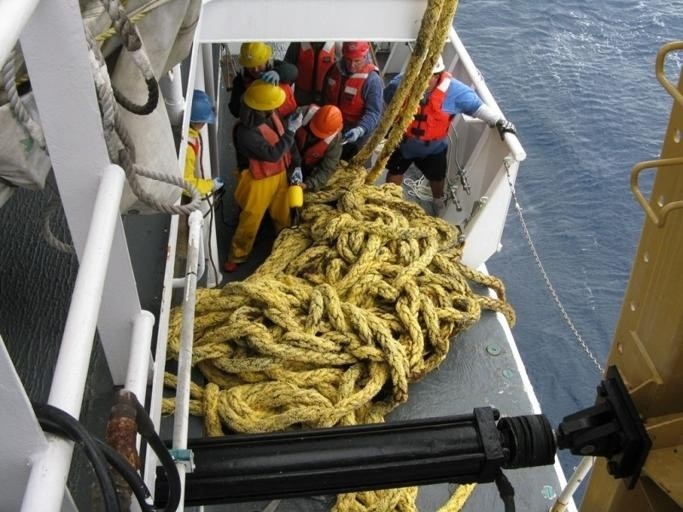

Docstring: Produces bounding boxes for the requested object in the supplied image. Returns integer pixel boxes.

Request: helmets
[243,80,286,111]
[190,90,215,124]
[309,105,343,139]
[432,55,446,74]
[238,41,271,67]
[342,41,369,57]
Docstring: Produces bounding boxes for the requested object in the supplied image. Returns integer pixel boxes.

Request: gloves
[291,166,302,185]
[213,178,224,192]
[287,109,303,134]
[263,71,279,86]
[345,126,364,143]
[496,118,517,142]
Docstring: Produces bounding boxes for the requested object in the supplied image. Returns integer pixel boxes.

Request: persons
[223,41,384,271]
[182,89,225,199]
[385,53,517,214]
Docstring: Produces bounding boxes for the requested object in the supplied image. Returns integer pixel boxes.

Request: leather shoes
[224,261,237,272]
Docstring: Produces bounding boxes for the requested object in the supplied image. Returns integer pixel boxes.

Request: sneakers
[432,197,444,213]
